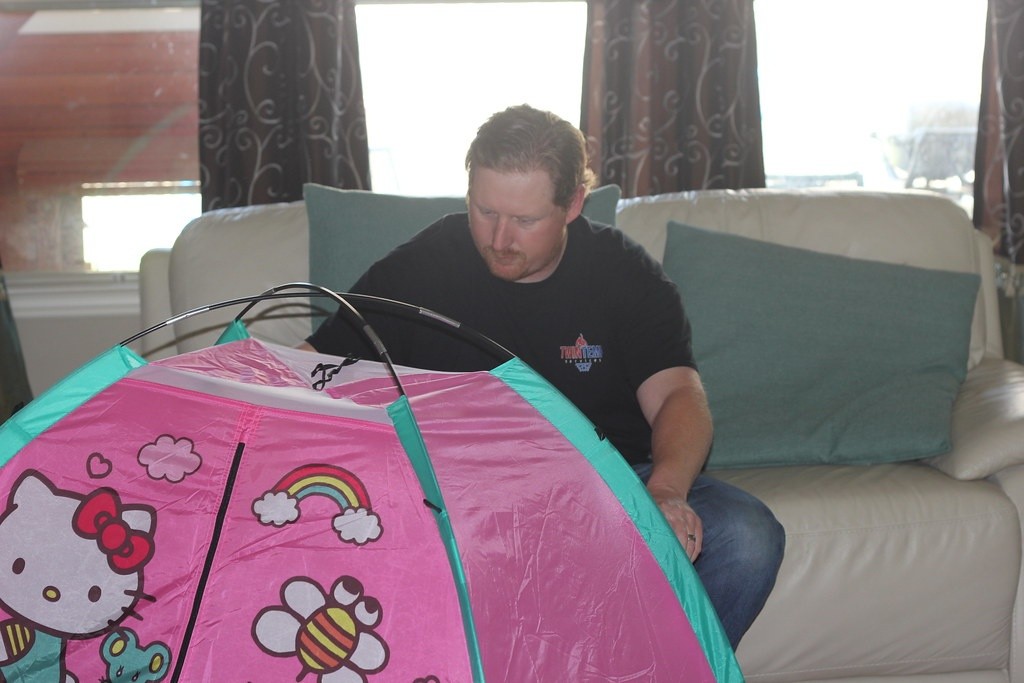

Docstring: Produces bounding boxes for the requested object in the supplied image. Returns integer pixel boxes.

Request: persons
[293,103,787,655]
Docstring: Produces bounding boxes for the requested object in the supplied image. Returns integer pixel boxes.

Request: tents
[0,282,746,683]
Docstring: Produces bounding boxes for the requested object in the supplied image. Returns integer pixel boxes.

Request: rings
[688,534,694,540]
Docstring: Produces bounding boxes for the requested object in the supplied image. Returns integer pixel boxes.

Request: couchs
[137,184,1024,683]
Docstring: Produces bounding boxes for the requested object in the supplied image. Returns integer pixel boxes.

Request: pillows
[302,184,621,338]
[658,222,981,469]
